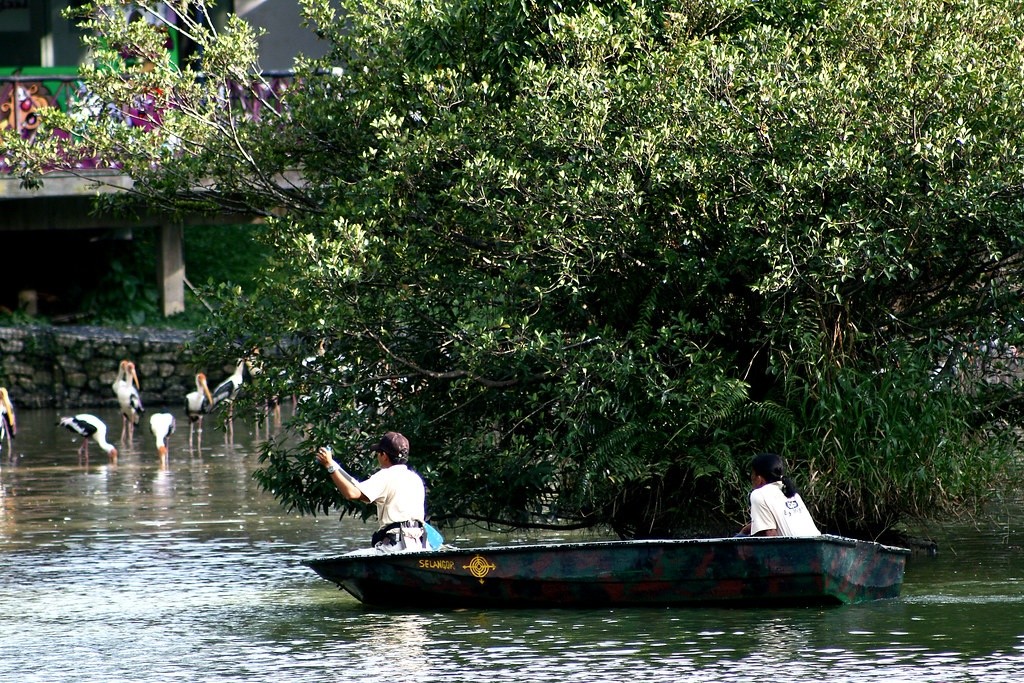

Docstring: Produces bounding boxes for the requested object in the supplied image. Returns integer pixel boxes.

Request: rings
[319,450,322,453]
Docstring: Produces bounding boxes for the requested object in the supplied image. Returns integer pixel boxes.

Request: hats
[370,431,409,458]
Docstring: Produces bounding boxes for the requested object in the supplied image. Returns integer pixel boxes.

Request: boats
[300,531,914,609]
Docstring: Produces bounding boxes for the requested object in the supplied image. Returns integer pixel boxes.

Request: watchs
[327,465,336,474]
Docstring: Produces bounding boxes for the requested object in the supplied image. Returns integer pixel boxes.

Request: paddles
[318,445,447,550]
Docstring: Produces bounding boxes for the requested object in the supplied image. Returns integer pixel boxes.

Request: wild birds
[54,413,117,462]
[111,360,145,441]
[150,412,176,460]
[0,387,16,439]
[183,355,302,433]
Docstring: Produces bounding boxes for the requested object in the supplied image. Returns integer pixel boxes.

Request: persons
[742,453,821,537]
[315,433,431,555]
